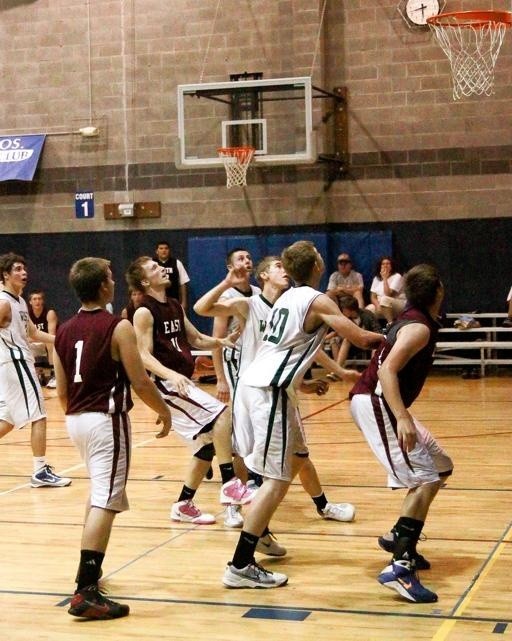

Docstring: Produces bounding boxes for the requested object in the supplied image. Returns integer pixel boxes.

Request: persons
[365,255,407,327]
[220,238,385,589]
[147,242,189,315]
[0,251,72,487]
[173,241,365,556]
[23,288,61,390]
[348,264,455,603]
[120,286,146,326]
[52,257,172,620]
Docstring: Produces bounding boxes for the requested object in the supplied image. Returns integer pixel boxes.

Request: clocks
[396,0,448,32]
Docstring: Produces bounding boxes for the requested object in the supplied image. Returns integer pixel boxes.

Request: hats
[336,253,352,262]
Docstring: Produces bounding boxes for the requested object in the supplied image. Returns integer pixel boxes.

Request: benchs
[186,348,216,359]
[320,313,512,375]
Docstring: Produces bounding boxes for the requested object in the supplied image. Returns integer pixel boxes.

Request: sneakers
[326,373,339,382]
[222,558,288,589]
[220,477,258,505]
[46,376,56,389]
[223,505,244,528]
[255,532,286,556]
[75,566,103,583]
[31,464,72,488]
[171,499,216,524]
[316,502,355,522]
[378,528,430,570]
[377,551,438,603]
[460,367,481,378]
[68,590,129,620]
